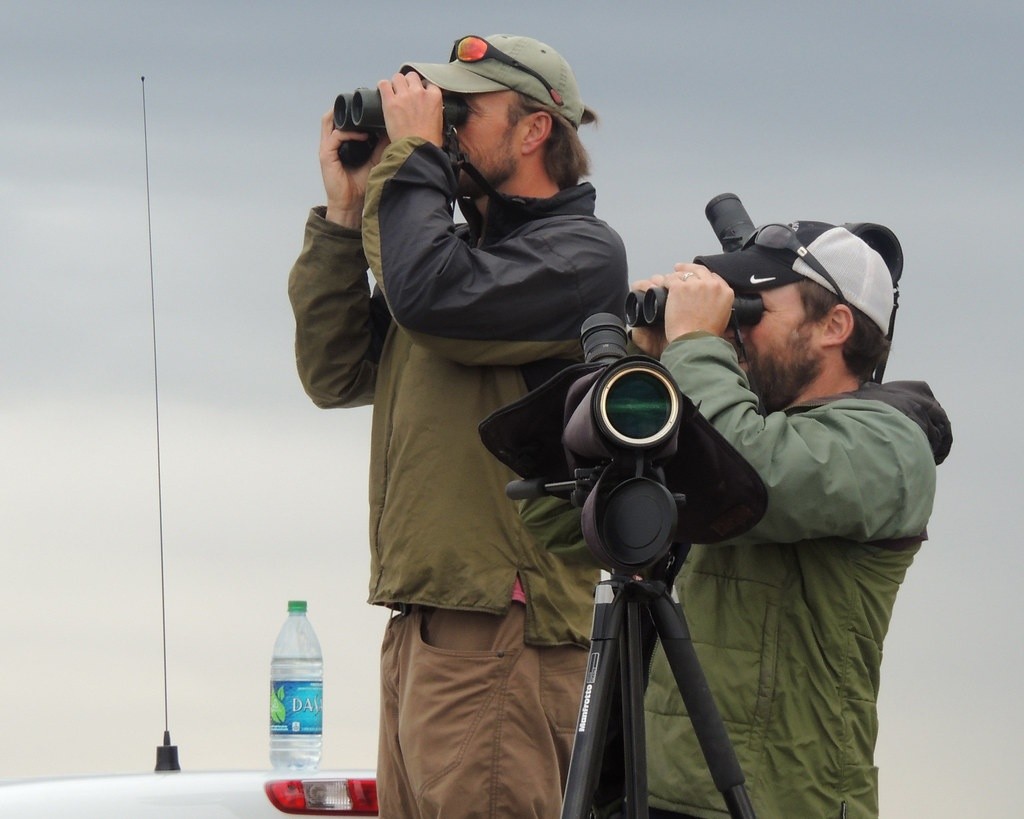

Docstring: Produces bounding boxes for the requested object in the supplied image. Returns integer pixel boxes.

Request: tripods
[504,460,756,819]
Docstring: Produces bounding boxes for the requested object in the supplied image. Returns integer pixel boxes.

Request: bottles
[270,601,323,777]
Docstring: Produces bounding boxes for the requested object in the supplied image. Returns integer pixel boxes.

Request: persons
[518,218,953,819]
[287,33,631,819]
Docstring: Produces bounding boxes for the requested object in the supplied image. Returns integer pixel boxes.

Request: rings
[681,271,695,281]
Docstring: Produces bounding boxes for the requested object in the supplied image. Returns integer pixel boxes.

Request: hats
[398,34,584,132]
[692,221,894,336]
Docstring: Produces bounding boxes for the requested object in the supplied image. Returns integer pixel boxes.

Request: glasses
[449,35,565,106]
[741,223,847,306]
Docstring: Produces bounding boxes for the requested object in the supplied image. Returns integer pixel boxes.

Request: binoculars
[623,286,765,329]
[333,88,466,131]
